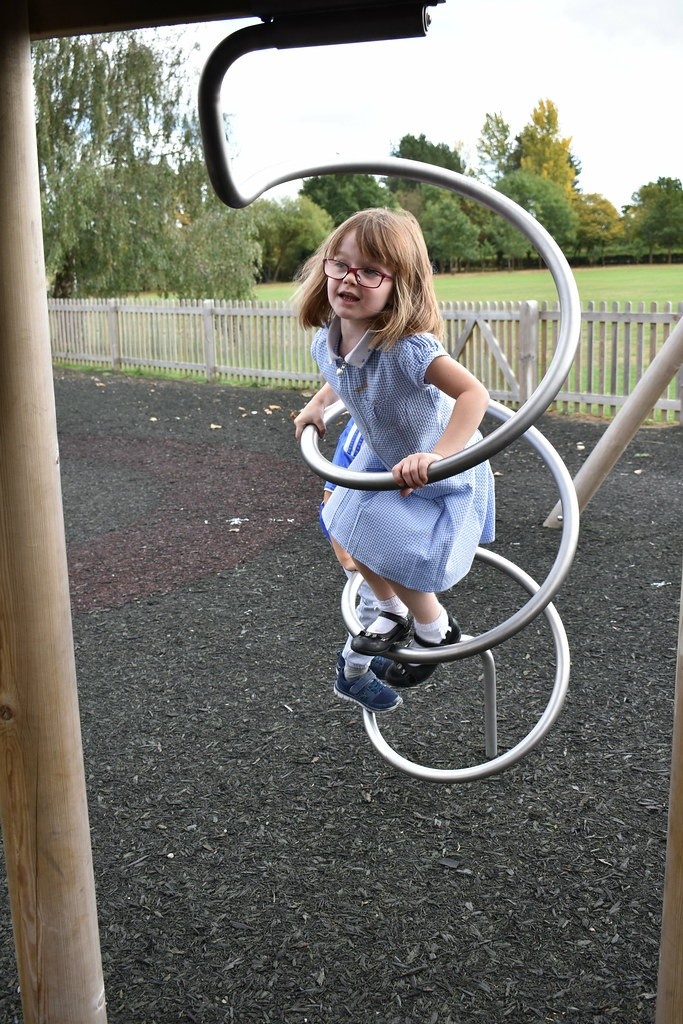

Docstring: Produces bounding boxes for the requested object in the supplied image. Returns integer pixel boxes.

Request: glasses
[320,256,396,290]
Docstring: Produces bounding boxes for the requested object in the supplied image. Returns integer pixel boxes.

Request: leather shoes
[387,616,461,686]
[350,610,412,657]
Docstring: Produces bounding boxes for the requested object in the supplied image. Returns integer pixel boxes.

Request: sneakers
[331,652,404,716]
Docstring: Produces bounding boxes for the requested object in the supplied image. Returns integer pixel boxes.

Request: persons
[294,206,495,713]
